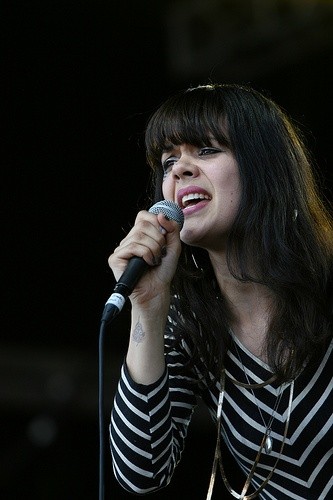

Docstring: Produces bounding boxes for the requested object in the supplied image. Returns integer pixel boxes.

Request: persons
[107,82,332,500]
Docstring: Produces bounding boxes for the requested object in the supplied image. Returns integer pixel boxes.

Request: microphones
[100,199,185,325]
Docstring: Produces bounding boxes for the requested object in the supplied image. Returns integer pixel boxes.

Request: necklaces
[206,316,296,499]
